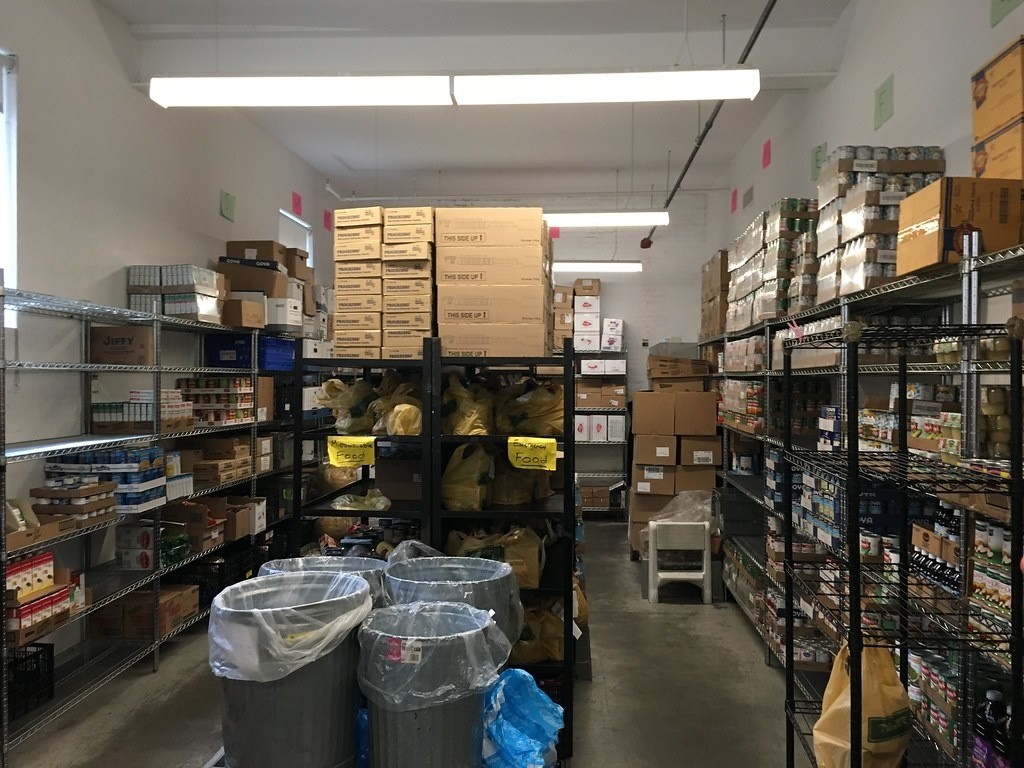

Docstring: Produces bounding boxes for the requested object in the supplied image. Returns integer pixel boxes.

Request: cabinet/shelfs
[698,230,1024,768]
[292,337,629,759]
[0,268,326,757]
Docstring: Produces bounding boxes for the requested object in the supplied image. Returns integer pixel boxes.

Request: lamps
[148,0,762,109]
[541,169,671,226]
[551,231,644,273]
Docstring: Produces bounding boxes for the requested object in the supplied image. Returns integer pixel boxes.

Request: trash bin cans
[381,556,513,671]
[210,555,388,768]
[357,601,489,768]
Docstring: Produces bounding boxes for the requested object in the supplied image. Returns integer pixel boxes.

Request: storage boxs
[5,206,625,728]
[915,709,959,758]
[628,34,1024,717]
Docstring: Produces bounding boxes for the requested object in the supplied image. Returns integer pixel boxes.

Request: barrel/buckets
[259,555,388,611]
[383,554,516,670]
[356,599,493,768]
[212,571,371,768]
